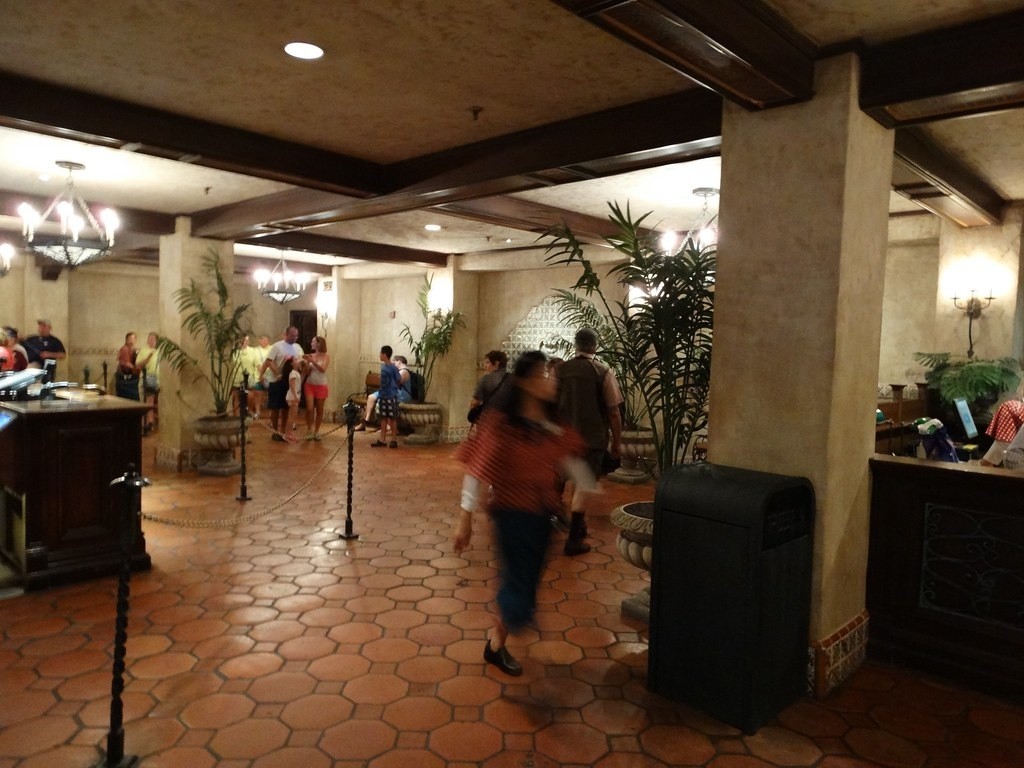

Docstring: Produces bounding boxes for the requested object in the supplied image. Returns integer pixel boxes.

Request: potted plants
[155,244,255,475]
[17,161,120,270]
[398,271,468,444]
[913,350,1022,416]
[539,287,662,485]
[535,200,719,622]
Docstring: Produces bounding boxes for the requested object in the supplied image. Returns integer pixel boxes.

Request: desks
[0,382,152,596]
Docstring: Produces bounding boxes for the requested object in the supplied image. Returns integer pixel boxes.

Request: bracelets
[313,363,316,365]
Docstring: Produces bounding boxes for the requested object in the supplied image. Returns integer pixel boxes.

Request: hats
[38,319,51,327]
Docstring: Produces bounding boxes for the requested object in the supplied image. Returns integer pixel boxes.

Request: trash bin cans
[646,462,817,736]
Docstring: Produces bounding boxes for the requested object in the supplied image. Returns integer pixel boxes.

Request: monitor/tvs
[0,368,48,390]
[952,397,979,447]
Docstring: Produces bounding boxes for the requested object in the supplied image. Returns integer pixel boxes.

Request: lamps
[0,244,14,277]
[253,250,310,303]
[659,188,720,256]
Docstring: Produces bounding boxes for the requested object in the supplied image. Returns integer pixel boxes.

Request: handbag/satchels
[467,404,487,424]
[146,374,156,392]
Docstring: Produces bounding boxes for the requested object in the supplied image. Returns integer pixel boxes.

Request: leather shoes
[484,640,522,676]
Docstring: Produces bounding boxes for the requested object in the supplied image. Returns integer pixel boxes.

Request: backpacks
[398,368,425,402]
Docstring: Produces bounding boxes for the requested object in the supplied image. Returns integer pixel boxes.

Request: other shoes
[283,433,299,443]
[254,412,260,418]
[390,441,397,448]
[353,423,365,431]
[377,424,391,433]
[272,432,277,439]
[314,433,321,441]
[276,434,282,441]
[564,541,591,557]
[371,440,387,447]
[306,433,312,440]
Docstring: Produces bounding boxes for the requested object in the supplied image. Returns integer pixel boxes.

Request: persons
[233,334,275,420]
[302,337,329,441]
[134,332,160,435]
[259,326,304,439]
[948,372,1024,470]
[370,345,403,449]
[21,319,66,385]
[280,355,301,444]
[0,326,28,378]
[353,356,425,432]
[448,327,624,678]
[114,332,140,403]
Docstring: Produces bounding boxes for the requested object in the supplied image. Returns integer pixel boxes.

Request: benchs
[346,371,382,411]
